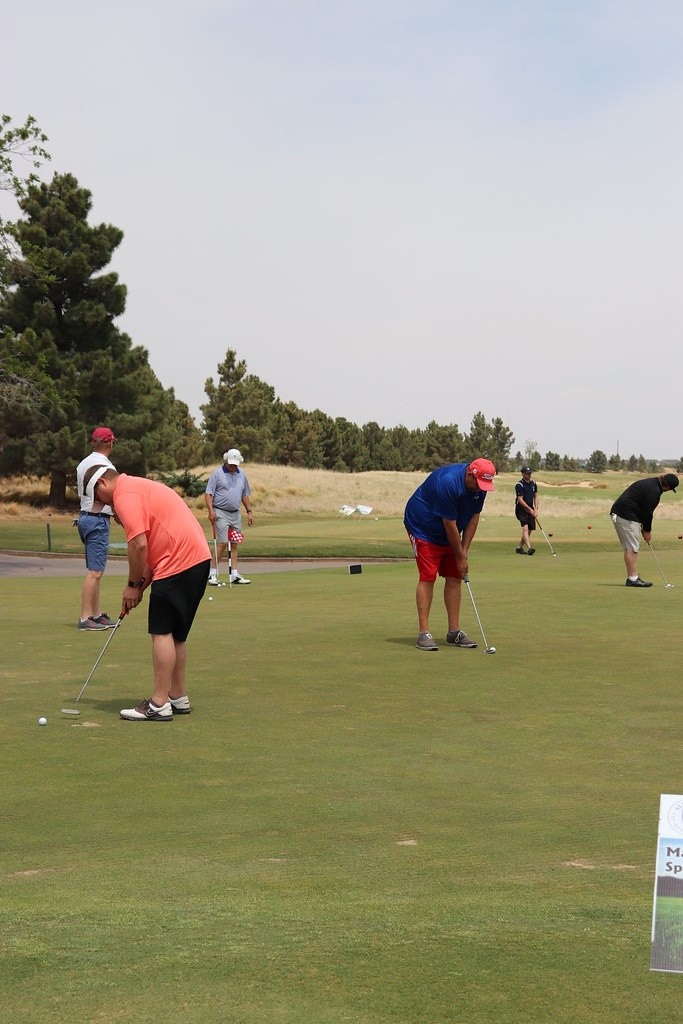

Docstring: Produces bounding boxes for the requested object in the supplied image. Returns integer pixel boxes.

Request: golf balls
[222,582,226,586]
[38,718,47,726]
[667,584,671,586]
[553,554,556,557]
[490,646,496,653]
[208,597,212,601]
[218,584,222,588]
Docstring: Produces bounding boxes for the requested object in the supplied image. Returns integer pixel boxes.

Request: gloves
[72,519,78,526]
[612,513,617,522]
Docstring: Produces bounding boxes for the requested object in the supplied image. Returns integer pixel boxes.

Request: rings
[131,604,135,606]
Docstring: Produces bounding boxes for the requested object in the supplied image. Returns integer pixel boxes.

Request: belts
[80,511,110,518]
[213,506,239,513]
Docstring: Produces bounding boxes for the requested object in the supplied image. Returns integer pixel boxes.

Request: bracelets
[535,506,538,509]
[247,510,252,513]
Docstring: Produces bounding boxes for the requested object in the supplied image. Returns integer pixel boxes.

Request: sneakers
[445,631,478,647]
[516,546,527,554]
[168,696,191,713]
[208,574,218,584]
[527,548,535,555]
[416,633,438,651]
[626,577,653,587]
[93,613,121,627]
[120,697,173,720]
[229,574,251,584]
[78,616,109,630]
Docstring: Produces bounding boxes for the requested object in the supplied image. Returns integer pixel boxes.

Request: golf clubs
[647,541,675,588]
[60,605,129,715]
[463,574,495,655]
[534,517,556,555]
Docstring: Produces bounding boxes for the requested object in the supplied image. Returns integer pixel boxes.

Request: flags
[228,529,244,543]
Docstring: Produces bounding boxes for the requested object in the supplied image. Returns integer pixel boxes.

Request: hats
[521,467,533,474]
[227,449,241,466]
[85,466,116,509]
[92,427,118,442]
[470,458,496,491]
[665,474,679,493]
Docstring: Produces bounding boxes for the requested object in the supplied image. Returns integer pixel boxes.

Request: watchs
[128,581,140,588]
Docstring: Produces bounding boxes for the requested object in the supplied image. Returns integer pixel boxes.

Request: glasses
[112,442,115,444]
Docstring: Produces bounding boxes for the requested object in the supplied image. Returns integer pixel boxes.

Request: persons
[403,458,496,649]
[72,427,121,631]
[515,468,539,555]
[82,464,212,722]
[609,474,680,587]
[205,449,254,585]
